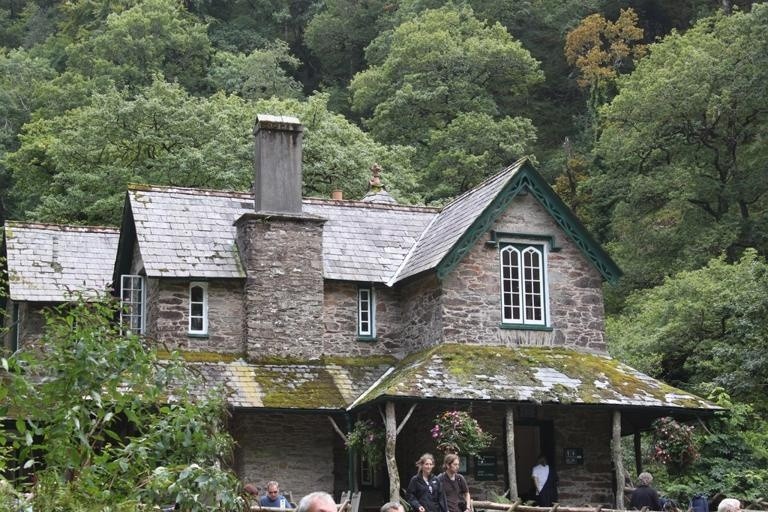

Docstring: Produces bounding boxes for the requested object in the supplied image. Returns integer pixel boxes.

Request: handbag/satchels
[458,501,476,512]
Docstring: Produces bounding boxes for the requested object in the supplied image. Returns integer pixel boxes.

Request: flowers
[345,419,388,467]
[650,416,698,473]
[431,410,495,465]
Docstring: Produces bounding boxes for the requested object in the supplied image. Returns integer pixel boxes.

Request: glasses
[267,491,279,493]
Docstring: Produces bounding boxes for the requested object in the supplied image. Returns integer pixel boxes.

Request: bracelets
[466,507,471,512]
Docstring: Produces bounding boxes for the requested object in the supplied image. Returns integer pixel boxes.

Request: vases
[668,461,679,474]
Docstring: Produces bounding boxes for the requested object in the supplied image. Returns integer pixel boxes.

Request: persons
[531,454,559,507]
[437,454,471,512]
[406,453,449,512]
[632,472,662,511]
[381,502,405,512]
[261,481,292,508]
[718,498,741,512]
[294,492,337,512]
